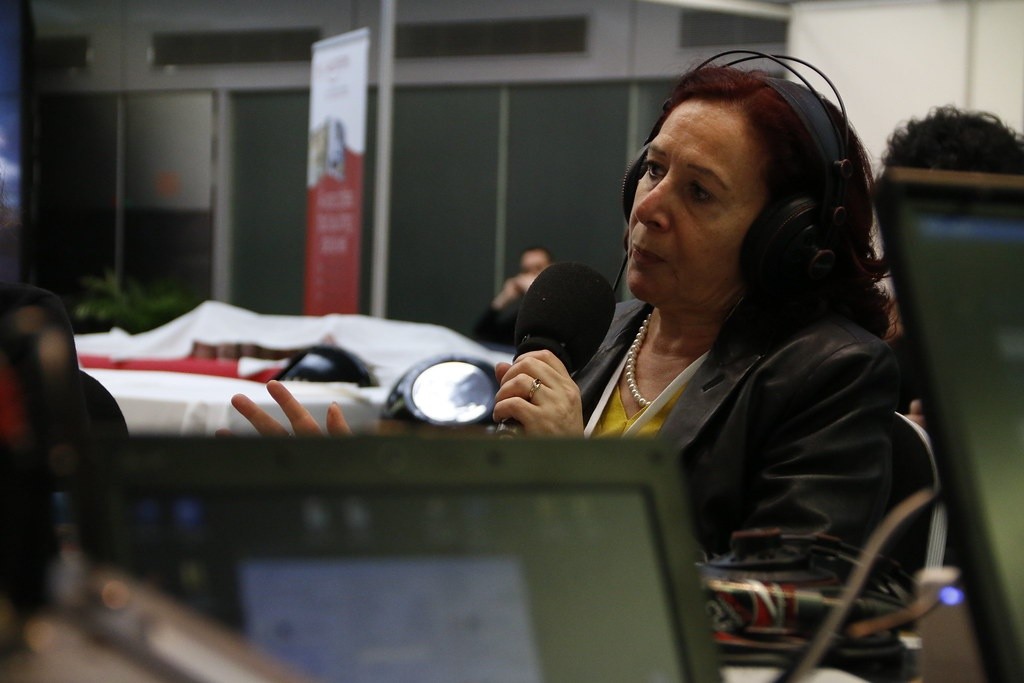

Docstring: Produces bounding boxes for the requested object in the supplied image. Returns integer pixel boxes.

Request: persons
[217,59,906,570]
[475,245,553,348]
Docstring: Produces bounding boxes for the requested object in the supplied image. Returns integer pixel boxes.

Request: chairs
[884,410,947,594]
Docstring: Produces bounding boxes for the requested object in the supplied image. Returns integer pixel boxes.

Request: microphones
[492,263,616,441]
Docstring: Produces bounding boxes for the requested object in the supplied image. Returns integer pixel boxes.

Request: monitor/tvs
[876,171,1024,683]
[74,427,724,683]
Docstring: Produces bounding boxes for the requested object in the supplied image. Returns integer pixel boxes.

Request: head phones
[696,519,920,683]
[620,50,857,310]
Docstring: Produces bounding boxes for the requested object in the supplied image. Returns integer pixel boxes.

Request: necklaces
[625,313,654,408]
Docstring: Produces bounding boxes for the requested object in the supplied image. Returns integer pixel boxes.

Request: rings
[528,378,541,403]
